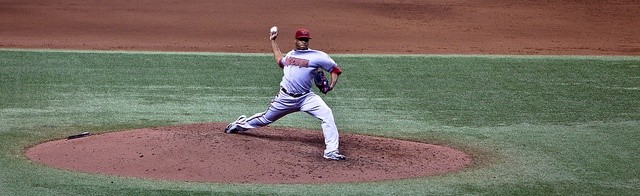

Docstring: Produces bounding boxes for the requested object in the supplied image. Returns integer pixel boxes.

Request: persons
[224,25,346,161]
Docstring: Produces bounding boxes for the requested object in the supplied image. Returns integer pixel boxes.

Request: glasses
[298,37,308,42]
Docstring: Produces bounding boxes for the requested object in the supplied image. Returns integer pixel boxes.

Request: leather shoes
[281,86,310,97]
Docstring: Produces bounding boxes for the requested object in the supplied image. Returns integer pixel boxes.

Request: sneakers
[323,151,346,160]
[224,115,246,133]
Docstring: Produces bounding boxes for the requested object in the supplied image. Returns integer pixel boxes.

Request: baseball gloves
[313,71,329,94]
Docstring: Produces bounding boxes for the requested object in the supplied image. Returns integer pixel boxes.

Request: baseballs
[270,26,278,33]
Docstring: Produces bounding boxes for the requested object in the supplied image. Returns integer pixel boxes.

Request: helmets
[295,28,312,39]
